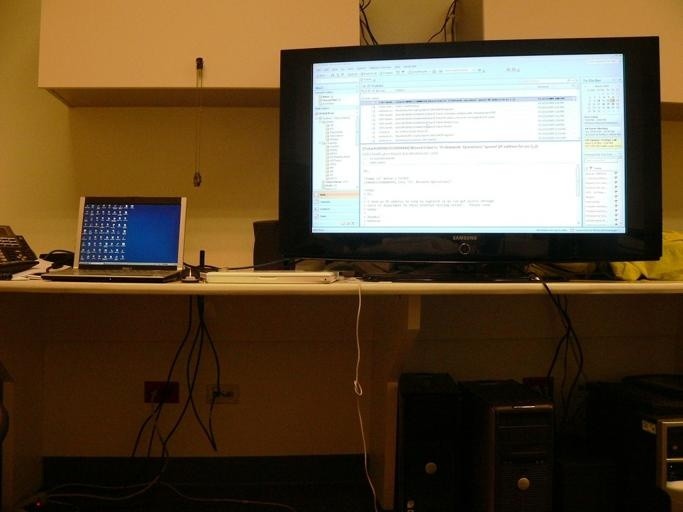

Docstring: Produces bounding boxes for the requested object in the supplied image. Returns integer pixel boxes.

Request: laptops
[205,269,341,284]
[41,198,188,282]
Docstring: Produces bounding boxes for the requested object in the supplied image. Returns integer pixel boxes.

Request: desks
[1,268,682,512]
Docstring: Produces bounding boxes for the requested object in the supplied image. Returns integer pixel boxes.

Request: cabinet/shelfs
[39,1,359,108]
[453,0,682,120]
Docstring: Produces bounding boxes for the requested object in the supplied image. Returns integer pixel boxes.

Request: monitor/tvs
[279,35,662,280]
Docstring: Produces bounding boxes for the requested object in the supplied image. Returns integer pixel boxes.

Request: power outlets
[204,384,242,405]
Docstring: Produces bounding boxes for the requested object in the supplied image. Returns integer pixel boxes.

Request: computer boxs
[395,372,466,510]
[586,387,683,511]
[467,379,557,509]
